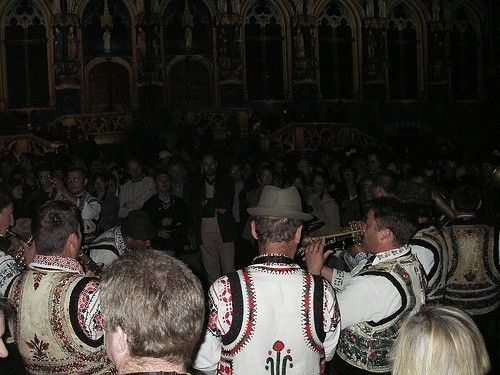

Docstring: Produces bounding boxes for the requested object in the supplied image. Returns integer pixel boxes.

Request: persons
[0,99,500,375]
[192,185,340,375]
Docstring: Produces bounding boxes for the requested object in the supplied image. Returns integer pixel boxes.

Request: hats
[5,175,23,193]
[121,210,158,240]
[158,149,174,160]
[246,185,313,221]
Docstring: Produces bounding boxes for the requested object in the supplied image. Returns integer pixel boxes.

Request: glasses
[37,174,53,179]
[94,180,106,186]
[66,177,85,184]
